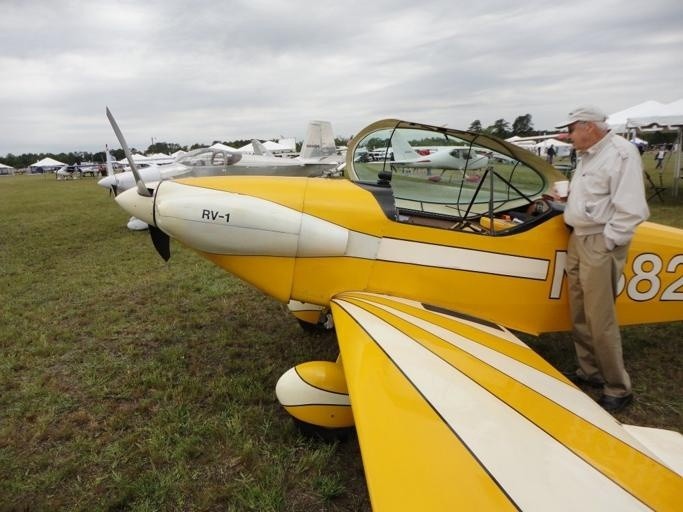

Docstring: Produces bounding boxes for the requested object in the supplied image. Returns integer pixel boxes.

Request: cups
[554,180,571,198]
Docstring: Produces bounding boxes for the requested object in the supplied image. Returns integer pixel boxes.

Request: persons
[637,143,644,156]
[654,147,667,168]
[555,105,650,413]
[546,144,555,165]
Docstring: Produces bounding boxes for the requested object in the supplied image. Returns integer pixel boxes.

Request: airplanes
[100,105,683,511]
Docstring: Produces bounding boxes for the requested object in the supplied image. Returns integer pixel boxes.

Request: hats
[554,106,607,129]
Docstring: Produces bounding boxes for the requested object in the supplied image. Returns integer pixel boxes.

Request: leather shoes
[596,394,633,413]
[567,372,604,388]
